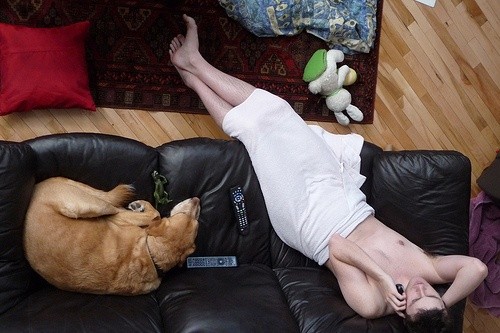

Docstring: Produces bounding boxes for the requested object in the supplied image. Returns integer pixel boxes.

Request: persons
[169,13,489,333]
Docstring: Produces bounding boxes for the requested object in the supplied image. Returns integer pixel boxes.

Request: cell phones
[396,284,404,302]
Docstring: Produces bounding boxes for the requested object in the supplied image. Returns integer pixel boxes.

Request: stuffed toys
[303,49,364,125]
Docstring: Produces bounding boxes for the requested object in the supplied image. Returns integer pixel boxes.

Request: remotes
[187,255,237,268]
[230,184,249,233]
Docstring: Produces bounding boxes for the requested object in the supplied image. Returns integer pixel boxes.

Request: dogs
[23,175,202,296]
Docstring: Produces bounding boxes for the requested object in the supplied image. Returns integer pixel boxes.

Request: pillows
[0,20,96,116]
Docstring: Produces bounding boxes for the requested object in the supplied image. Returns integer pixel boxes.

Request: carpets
[0,0,383,124]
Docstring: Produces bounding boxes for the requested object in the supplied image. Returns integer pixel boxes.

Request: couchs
[0,132,473,333]
